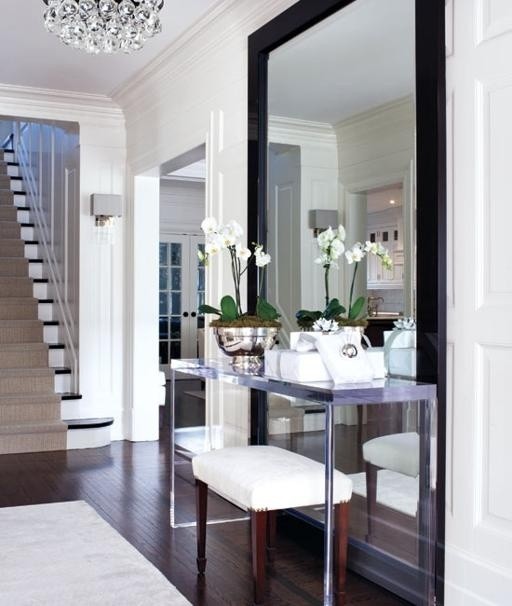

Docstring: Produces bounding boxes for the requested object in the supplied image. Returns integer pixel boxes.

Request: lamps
[90,193,122,227]
[309,208,338,237]
[42,0,164,54]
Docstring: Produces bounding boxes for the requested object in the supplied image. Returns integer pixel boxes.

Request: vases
[299,319,368,355]
[212,318,282,366]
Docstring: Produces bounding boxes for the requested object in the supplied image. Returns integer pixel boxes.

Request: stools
[189,445,353,606]
[362,431,419,536]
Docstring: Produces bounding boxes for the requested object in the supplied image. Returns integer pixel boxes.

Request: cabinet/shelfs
[367,227,404,282]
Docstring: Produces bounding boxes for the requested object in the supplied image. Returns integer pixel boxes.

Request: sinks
[374,311,400,317]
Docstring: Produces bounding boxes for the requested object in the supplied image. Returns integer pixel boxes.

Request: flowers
[295,223,393,327]
[194,218,282,322]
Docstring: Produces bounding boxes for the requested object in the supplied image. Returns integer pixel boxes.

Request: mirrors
[246,1,440,603]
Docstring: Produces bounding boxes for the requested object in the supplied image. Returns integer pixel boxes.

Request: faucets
[367,297,385,303]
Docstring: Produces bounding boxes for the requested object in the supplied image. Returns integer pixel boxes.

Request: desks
[167,359,441,606]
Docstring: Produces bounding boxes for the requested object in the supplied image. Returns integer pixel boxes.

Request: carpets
[344,470,422,517]
[0,500,192,606]
